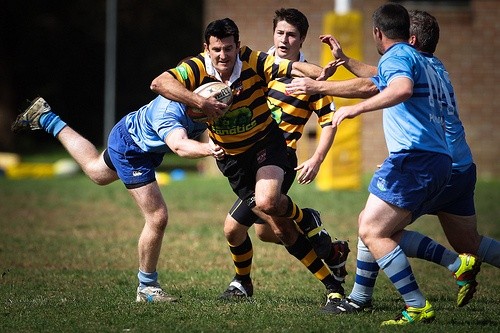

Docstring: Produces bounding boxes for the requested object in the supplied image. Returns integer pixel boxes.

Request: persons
[224,8,350,298]
[9,57,226,304]
[332,2,483,323]
[151,18,347,312]
[285,10,499,316]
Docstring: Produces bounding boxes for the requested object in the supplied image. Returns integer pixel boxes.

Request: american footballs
[186,81,233,122]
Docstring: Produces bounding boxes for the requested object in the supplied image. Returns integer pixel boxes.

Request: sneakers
[219,278,253,300]
[297,208,332,260]
[324,240,351,284]
[137,284,182,303]
[325,286,344,306]
[321,298,373,315]
[380,298,436,326]
[450,253,482,307]
[10,96,51,132]
